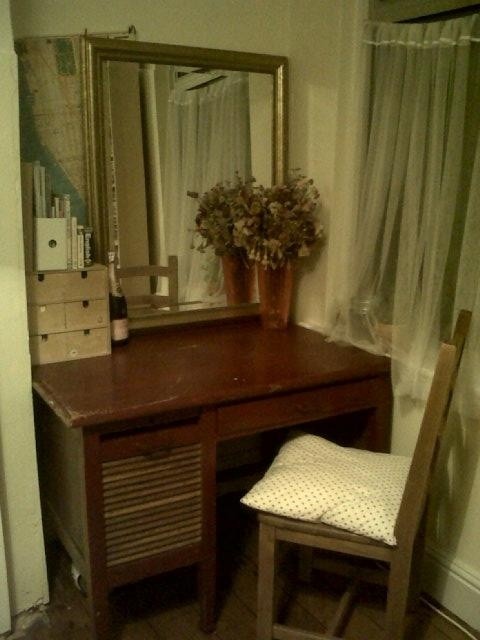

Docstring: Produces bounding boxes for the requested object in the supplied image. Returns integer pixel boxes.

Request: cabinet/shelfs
[28,263,112,368]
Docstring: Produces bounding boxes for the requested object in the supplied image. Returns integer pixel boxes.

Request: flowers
[184,177,252,270]
[232,166,329,275]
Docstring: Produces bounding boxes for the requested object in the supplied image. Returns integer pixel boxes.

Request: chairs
[237,307,474,639]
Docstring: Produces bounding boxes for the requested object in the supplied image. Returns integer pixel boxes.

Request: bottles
[108,256,130,347]
[348,292,384,347]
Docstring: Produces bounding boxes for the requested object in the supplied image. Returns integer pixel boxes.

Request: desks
[32,315,395,639]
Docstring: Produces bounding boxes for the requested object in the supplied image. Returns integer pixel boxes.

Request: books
[33,163,94,271]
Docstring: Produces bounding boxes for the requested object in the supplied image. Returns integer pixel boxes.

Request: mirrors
[78,32,290,333]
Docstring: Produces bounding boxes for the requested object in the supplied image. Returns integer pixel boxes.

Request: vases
[221,254,257,306]
[257,258,295,332]
